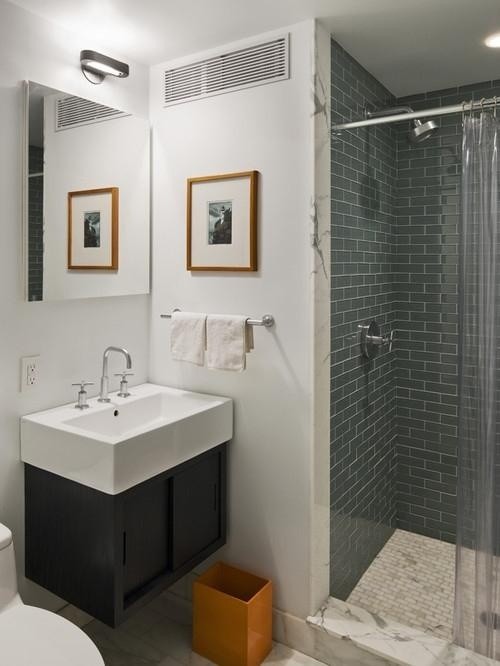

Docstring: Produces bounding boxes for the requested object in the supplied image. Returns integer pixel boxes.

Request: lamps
[79,49,130,86]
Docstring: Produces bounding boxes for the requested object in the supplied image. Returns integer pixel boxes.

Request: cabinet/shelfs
[122,450,223,610]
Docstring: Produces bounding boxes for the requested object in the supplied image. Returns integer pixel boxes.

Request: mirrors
[17,78,153,303]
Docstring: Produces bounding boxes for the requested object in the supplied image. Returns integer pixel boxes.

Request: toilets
[0,523,106,666]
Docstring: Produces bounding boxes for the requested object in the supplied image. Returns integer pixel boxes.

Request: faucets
[98,345,131,403]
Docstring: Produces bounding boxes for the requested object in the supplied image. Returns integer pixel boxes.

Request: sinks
[20,382,234,496]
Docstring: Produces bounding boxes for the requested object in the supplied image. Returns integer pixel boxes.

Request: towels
[169,311,255,372]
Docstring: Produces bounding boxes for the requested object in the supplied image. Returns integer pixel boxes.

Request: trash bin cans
[191,560,273,666]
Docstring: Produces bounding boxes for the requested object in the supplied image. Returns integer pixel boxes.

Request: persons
[88,215,93,231]
[219,205,226,224]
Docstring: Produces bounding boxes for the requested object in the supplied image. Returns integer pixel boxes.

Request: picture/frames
[185,169,260,272]
[66,186,119,272]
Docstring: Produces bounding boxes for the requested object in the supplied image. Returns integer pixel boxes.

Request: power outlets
[19,355,42,394]
